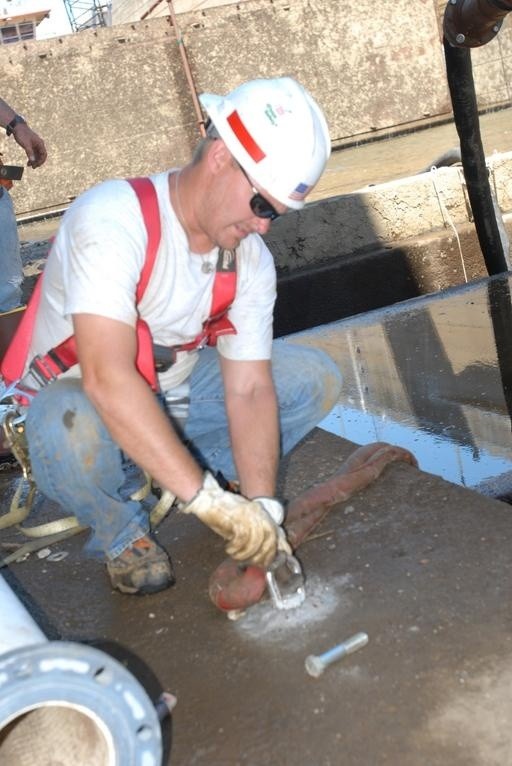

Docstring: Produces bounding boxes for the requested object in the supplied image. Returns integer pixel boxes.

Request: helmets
[198,77,331,211]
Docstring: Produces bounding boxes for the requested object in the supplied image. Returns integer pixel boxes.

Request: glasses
[235,160,280,221]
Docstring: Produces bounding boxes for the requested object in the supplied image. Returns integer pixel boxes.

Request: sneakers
[105,535,175,595]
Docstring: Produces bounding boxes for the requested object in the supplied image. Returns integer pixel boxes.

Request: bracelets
[4,114,28,138]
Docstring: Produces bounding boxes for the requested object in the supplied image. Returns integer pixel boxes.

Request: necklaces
[171,168,219,276]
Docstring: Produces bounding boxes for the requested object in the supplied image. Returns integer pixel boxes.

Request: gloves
[178,470,292,565]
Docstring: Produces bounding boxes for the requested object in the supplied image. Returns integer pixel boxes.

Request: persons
[0,92,47,314]
[1,76,345,603]
